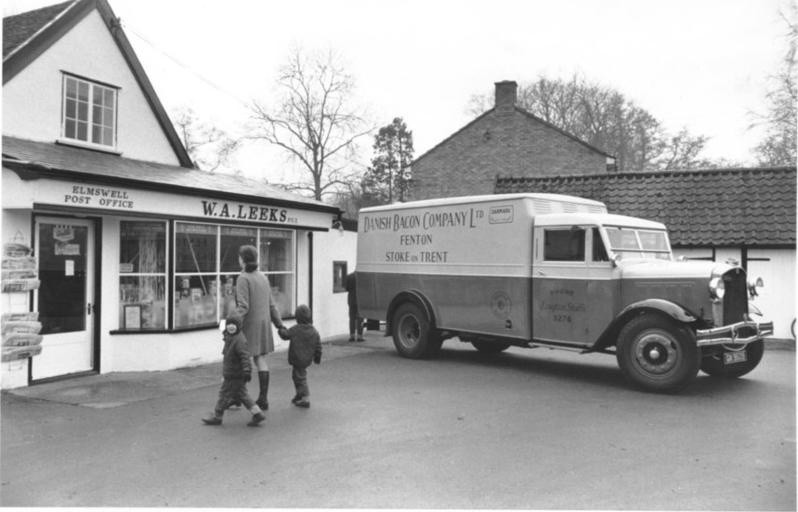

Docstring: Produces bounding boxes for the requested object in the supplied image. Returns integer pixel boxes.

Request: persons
[344,270,368,343]
[277,302,323,409]
[227,244,287,412]
[198,312,268,427]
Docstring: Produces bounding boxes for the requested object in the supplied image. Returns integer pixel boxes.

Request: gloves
[314,355,320,365]
[242,373,252,382]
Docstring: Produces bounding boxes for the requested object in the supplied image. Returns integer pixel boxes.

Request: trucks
[355,189,775,396]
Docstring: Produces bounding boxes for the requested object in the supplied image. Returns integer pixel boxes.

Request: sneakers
[248,412,265,427]
[202,417,222,424]
[349,337,365,342]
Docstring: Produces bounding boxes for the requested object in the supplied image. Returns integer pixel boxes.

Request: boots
[291,396,310,407]
[255,371,269,409]
